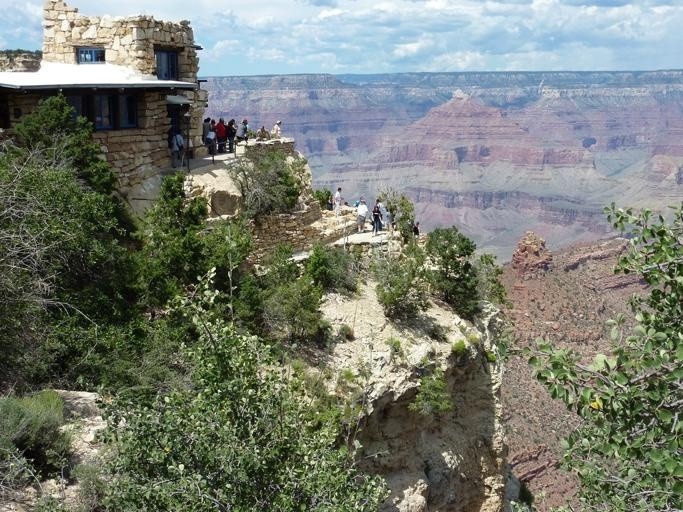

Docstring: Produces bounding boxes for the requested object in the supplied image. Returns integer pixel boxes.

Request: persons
[255,125,271,142]
[270,120,281,139]
[202,117,248,155]
[370,206,383,237]
[332,187,342,218]
[356,198,367,234]
[170,129,187,169]
[413,221,419,239]
[374,199,387,225]
